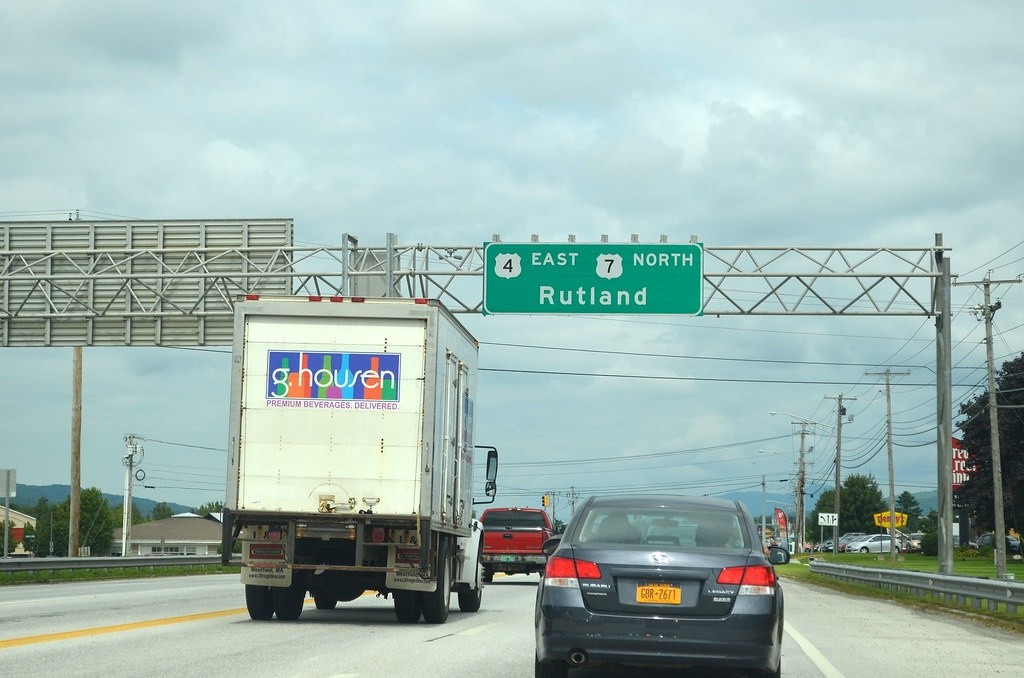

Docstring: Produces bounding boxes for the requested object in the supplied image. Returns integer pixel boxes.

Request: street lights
[757,449,805,554]
[771,411,841,556]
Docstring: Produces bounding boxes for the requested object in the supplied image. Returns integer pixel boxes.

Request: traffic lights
[541,496,548,507]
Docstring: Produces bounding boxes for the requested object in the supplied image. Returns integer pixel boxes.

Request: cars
[533,491,791,678]
[977,533,1021,558]
[805,532,980,554]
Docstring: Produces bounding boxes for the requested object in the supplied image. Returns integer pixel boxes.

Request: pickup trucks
[477,506,566,582]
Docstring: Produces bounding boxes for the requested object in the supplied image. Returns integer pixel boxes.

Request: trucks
[221,296,499,624]
